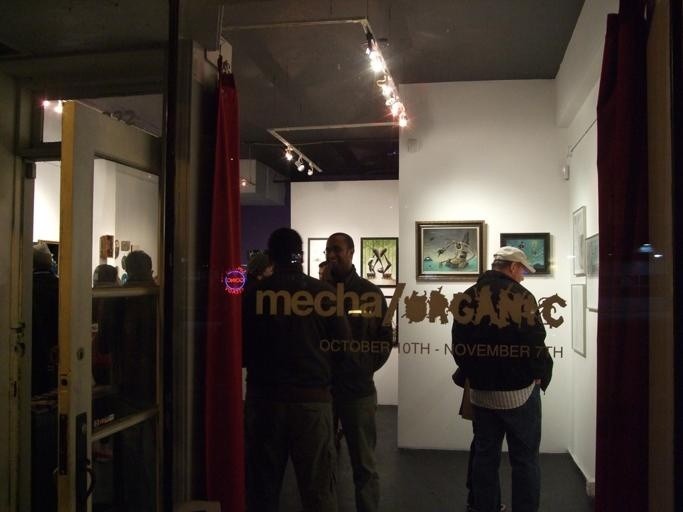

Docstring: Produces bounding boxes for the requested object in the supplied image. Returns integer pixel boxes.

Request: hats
[493,246,537,273]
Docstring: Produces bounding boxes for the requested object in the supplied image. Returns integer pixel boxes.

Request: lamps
[283,145,316,180]
[362,32,410,133]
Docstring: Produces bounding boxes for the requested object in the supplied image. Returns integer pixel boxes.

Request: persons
[449,244,553,512]
[320,231,393,512]
[318,261,344,453]
[31,237,161,510]
[240,227,362,512]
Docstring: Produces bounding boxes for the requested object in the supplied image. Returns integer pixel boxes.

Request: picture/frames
[305,236,329,279]
[569,205,598,357]
[359,237,398,290]
[413,221,485,280]
[499,232,551,275]
[383,296,399,350]
